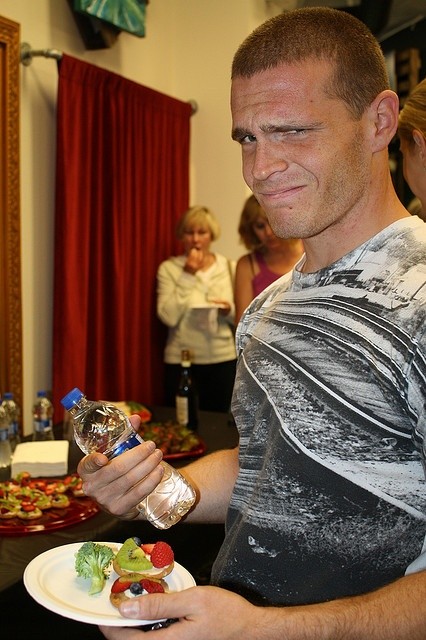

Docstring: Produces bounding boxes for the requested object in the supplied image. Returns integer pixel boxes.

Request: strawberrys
[140,579,164,594]
[111,575,130,594]
[141,544,156,554]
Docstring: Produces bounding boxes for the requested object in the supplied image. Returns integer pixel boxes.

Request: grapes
[132,537,141,546]
[130,583,142,595]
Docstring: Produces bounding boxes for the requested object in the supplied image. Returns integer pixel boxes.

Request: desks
[0,402,237,592]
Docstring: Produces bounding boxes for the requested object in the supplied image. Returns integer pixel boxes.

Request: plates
[137,431,206,459]
[0,499,102,537]
[191,303,228,307]
[23,540,199,627]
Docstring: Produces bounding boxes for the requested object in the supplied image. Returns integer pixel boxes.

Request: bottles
[60,388,197,532]
[174,349,200,430]
[0,393,21,471]
[32,389,56,445]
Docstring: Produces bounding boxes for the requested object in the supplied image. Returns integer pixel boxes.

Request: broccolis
[75,541,114,595]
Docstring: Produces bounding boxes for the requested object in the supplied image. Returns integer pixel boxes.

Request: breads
[110,573,170,611]
[0,473,107,521]
[111,559,175,582]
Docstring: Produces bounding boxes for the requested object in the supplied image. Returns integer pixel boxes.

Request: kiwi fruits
[116,539,153,570]
[0,470,83,518]
[119,573,147,584]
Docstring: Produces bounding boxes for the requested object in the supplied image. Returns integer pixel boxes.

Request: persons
[231,193,302,325]
[78,6,425,637]
[158,206,237,410]
[397,79,426,207]
